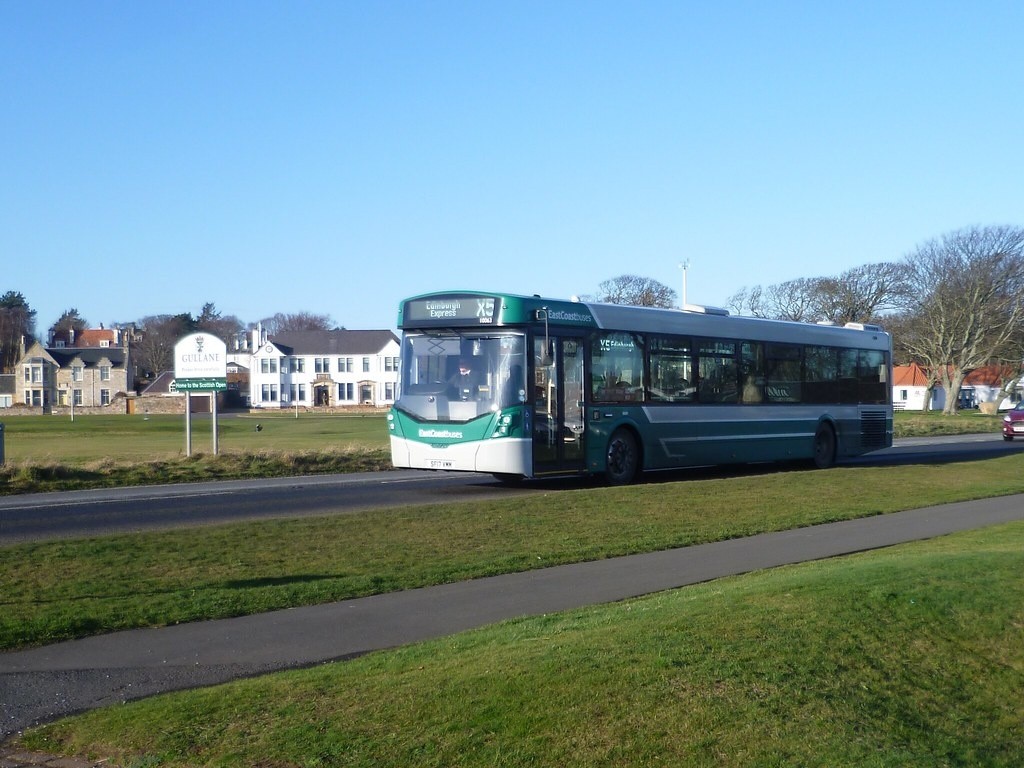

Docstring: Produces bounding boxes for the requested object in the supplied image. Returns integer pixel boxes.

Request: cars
[1001,399,1024,441]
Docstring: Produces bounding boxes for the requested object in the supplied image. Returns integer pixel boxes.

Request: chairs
[667,359,871,402]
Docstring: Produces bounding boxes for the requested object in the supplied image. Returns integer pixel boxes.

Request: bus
[383,290,894,486]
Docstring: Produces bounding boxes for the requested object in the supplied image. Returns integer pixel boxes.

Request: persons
[445,356,483,397]
